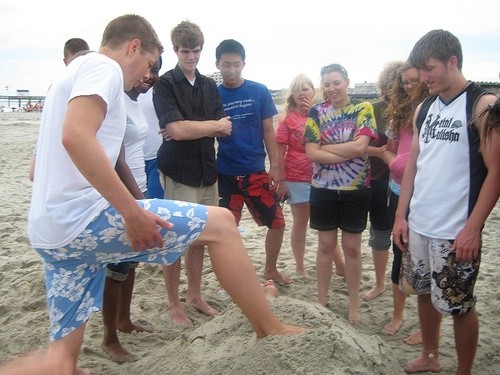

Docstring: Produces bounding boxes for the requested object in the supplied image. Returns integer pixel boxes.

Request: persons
[29,13,305,375]
[64,39,166,362]
[361,62,405,301]
[213,38,290,291]
[381,62,429,345]
[26,99,42,110]
[277,75,349,279]
[152,21,231,328]
[303,65,378,324]
[392,29,500,375]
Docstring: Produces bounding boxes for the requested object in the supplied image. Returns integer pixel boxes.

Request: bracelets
[280,179,286,181]
